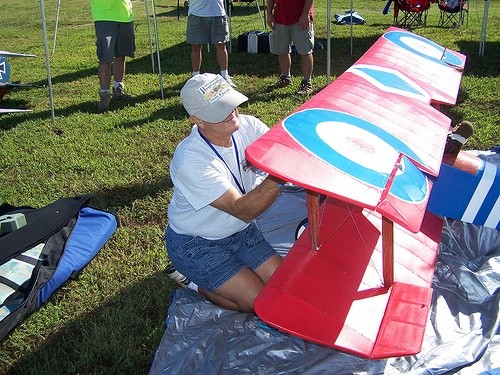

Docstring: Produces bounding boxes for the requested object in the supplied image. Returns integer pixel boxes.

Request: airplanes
[244,26,500,362]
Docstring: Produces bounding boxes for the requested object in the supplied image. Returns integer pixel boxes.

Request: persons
[165,73,288,313]
[91,0,136,111]
[185,0,237,89]
[266,0,315,96]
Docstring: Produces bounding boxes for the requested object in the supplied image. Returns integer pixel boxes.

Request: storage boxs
[238,29,269,53]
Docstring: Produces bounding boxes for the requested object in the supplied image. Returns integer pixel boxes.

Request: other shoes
[224,76,237,88]
[97,91,110,112]
[163,260,191,287]
[112,84,132,102]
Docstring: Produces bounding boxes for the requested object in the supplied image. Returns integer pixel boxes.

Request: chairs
[438,0,470,30]
[383,0,439,31]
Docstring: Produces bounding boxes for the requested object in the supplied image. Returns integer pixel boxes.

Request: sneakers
[267,75,292,92]
[296,80,313,96]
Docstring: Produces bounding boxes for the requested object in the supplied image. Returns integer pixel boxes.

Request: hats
[180,73,249,124]
